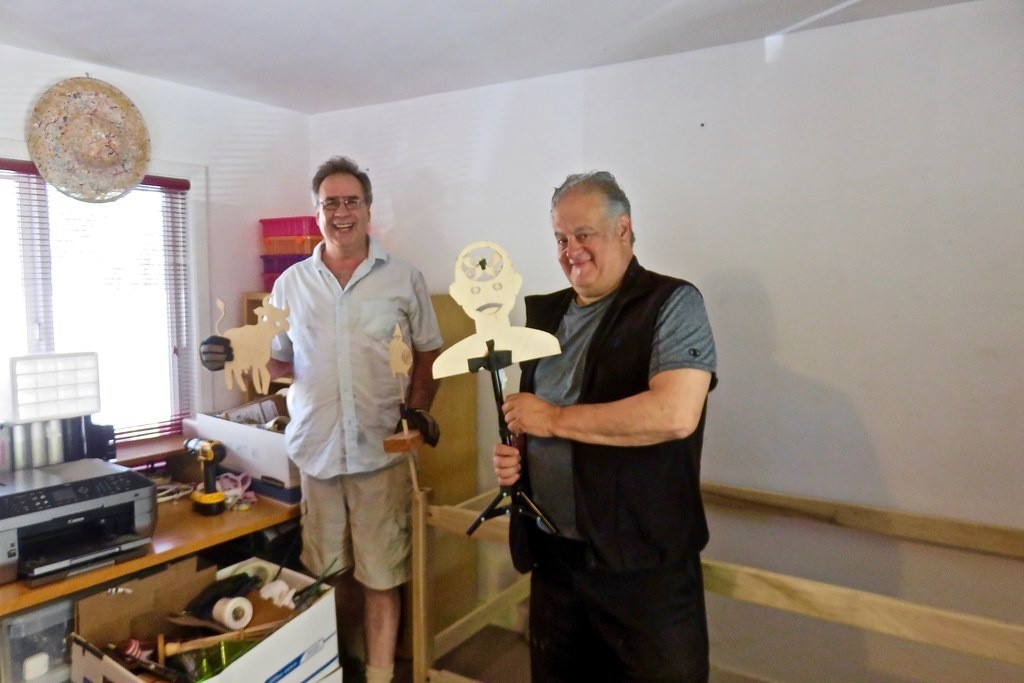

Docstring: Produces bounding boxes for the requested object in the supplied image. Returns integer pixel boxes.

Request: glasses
[320,199,366,211]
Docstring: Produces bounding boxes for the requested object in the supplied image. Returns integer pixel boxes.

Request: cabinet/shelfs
[244,290,480,661]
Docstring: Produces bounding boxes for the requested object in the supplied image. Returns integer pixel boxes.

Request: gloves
[407,409,440,448]
[199,335,235,371]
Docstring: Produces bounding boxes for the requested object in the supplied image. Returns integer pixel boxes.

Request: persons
[490,169,718,683]
[199,156,446,683]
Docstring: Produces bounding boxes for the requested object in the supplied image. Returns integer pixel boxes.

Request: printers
[0,458,158,587]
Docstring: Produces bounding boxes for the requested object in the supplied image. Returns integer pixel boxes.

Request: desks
[0,482,302,616]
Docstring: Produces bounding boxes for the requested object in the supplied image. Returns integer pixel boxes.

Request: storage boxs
[195,394,302,508]
[10,600,72,683]
[259,216,324,293]
[72,556,344,683]
